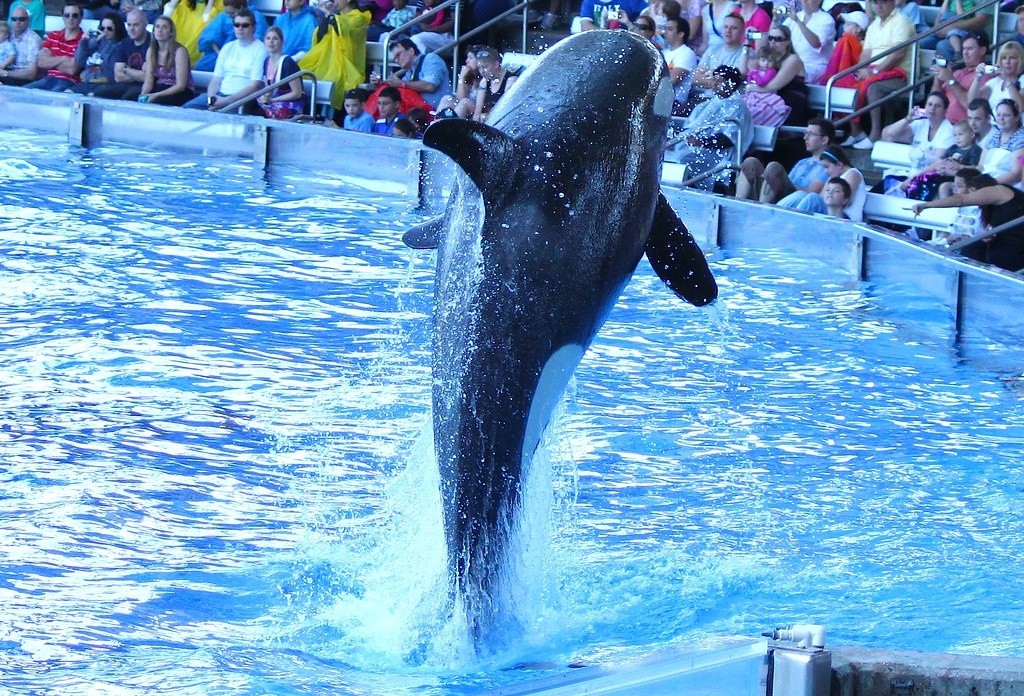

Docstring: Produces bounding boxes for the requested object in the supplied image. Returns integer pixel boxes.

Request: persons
[244,26,306,120]
[0,0,517,140]
[20,0,84,92]
[577,0,1024,273]
[524,0,564,32]
[181,9,271,115]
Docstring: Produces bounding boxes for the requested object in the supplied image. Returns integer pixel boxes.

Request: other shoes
[852,137,875,149]
[540,12,563,31]
[841,130,868,146]
[521,10,544,23]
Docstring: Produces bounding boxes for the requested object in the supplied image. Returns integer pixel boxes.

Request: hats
[967,29,992,55]
[841,11,869,30]
[436,107,457,119]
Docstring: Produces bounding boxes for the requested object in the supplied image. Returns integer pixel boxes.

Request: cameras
[983,65,999,74]
[777,6,787,14]
[608,10,619,19]
[374,75,381,84]
[138,95,149,103]
[208,96,216,105]
[933,57,947,67]
[746,29,762,40]
[90,31,100,38]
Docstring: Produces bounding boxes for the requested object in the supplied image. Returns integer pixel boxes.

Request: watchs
[871,67,879,76]
[947,79,957,86]
[458,79,465,84]
[742,48,748,55]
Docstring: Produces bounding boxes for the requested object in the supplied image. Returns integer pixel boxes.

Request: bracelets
[1006,81,1013,89]
[4,70,8,77]
[743,43,751,48]
[123,65,128,74]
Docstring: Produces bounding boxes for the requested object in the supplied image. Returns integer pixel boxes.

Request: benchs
[1,0,983,259]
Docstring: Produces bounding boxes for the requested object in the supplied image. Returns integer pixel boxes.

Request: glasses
[473,45,482,53]
[10,16,29,22]
[393,49,409,63]
[768,35,788,42]
[63,12,81,18]
[233,21,253,28]
[804,129,825,137]
[476,51,497,60]
[634,22,652,31]
[951,184,972,189]
[98,24,117,31]
